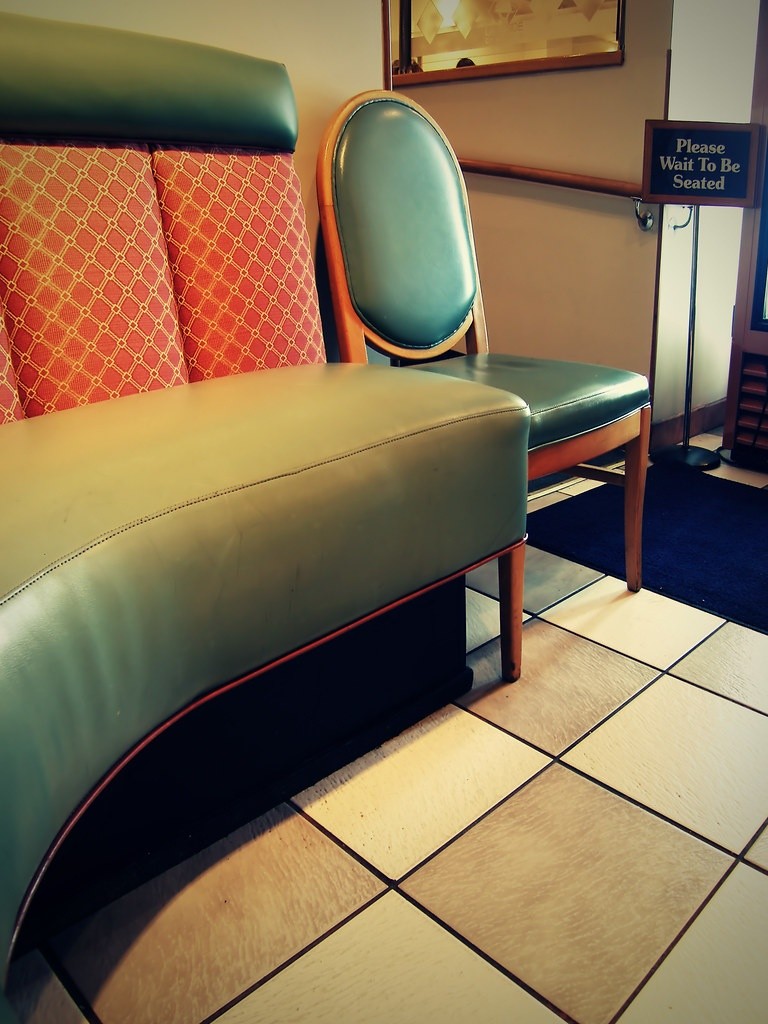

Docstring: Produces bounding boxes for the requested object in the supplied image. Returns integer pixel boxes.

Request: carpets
[524,464,768,637]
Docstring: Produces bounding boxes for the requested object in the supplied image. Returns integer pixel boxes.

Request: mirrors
[380,0,627,92]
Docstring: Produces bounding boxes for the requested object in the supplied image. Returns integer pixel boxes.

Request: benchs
[0,14,531,1011]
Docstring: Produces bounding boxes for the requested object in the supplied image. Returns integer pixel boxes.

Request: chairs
[316,88,652,685]
